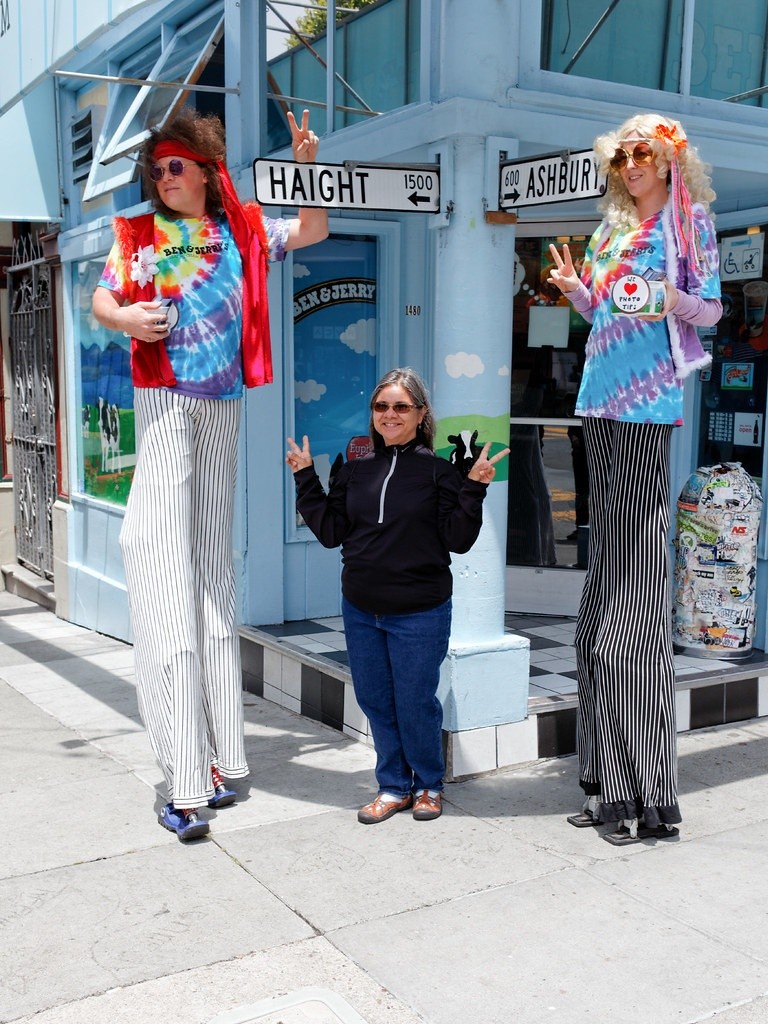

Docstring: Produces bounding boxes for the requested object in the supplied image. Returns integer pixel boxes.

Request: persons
[546,112,723,667]
[285,368,511,824]
[92,110,329,663]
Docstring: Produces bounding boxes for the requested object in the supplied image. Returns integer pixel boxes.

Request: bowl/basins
[609,280,666,317]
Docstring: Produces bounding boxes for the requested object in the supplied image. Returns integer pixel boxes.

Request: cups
[743,281,768,338]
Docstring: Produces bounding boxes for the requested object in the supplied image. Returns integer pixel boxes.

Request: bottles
[753,420,758,444]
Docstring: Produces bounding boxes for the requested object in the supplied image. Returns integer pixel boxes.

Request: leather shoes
[413,789,441,819]
[358,793,412,823]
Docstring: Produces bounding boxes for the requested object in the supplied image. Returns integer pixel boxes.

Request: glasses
[371,401,416,414]
[147,159,200,183]
[608,143,654,171]
